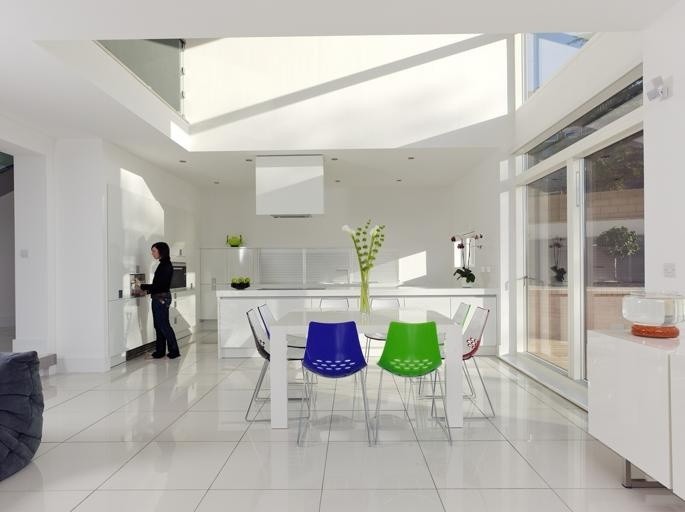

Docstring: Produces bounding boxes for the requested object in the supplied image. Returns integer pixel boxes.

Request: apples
[232,276,251,283]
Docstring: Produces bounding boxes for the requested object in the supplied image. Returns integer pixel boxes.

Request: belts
[150,292,169,305]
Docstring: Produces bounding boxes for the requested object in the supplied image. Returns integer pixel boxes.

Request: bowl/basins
[230,281,250,290]
[619,290,684,326]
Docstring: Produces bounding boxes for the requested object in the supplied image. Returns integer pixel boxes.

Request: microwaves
[167,262,186,288]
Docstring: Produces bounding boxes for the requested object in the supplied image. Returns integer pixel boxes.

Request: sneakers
[151,351,166,358]
[167,352,180,358]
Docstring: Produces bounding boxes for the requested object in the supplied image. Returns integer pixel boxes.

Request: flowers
[451,230,484,283]
[548,232,568,282]
[342,217,387,317]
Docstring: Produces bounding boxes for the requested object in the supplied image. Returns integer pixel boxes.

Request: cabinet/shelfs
[198,245,258,323]
[584,325,685,503]
[166,287,197,349]
[213,286,266,361]
[124,293,159,361]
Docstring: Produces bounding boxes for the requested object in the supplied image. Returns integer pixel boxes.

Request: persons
[134,243,181,360]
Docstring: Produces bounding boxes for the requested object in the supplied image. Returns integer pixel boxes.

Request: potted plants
[595,223,641,284]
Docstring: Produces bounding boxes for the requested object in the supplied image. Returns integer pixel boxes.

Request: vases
[358,281,371,326]
[461,276,474,289]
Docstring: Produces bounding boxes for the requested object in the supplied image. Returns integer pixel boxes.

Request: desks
[269,309,463,427]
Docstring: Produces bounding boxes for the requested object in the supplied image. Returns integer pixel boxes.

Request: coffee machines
[128,273,145,297]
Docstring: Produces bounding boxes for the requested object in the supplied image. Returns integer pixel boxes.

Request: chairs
[0,349,46,481]
[420,303,496,419]
[244,292,318,422]
[374,322,453,446]
[297,321,373,446]
